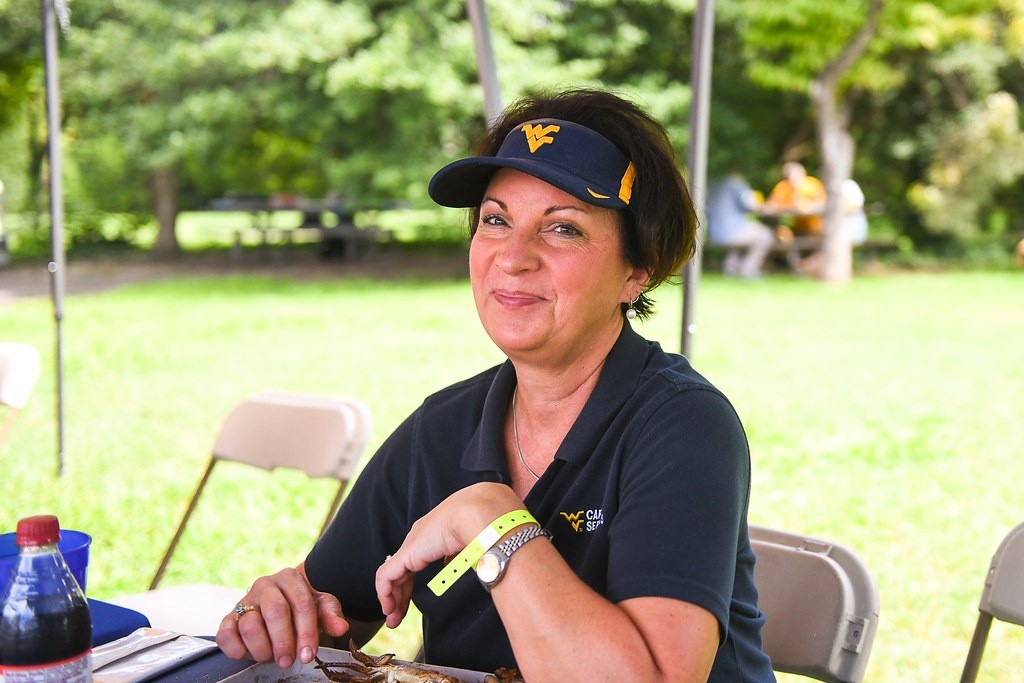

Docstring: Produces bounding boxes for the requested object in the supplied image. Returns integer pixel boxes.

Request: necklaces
[512,383,539,480]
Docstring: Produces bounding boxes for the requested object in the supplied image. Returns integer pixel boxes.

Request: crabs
[311,639,520,683]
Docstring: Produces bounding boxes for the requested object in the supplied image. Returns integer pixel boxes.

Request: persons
[215,86,778,683]
[707,161,869,279]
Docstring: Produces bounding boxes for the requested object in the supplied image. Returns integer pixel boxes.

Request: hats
[428,117,634,214]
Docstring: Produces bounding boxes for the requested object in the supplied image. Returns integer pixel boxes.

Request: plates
[213,645,495,683]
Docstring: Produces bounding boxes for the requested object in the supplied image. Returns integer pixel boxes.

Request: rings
[233,603,258,622]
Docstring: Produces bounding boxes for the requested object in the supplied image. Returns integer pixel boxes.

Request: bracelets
[426,510,541,597]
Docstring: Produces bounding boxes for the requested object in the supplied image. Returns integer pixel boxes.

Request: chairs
[960,521,1024,683]
[748,526,883,683]
[99,391,374,636]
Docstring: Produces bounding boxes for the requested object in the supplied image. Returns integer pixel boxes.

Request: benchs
[772,237,900,273]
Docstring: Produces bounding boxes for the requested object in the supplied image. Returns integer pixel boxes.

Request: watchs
[475,525,554,592]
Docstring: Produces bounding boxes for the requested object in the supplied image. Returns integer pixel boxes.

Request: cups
[0,528,92,607]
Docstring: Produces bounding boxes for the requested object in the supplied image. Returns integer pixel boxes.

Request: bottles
[0,515,96,683]
